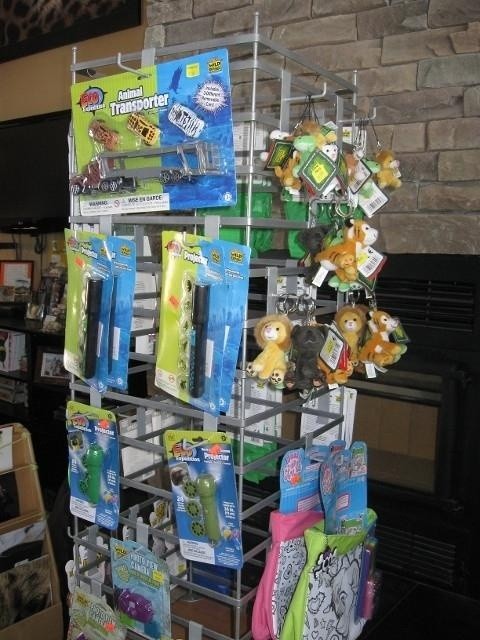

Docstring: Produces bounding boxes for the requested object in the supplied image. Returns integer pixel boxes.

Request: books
[0,425,54,632]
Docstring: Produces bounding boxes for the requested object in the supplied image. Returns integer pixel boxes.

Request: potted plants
[0,260,34,306]
[34,344,76,386]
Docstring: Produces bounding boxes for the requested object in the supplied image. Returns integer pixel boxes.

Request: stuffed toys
[334,305,366,366]
[290,325,328,388]
[260,130,288,162]
[376,150,401,189]
[357,311,407,367]
[315,218,378,282]
[273,121,363,195]
[246,314,293,390]
[294,224,336,284]
[358,159,380,199]
[317,357,353,384]
[327,230,363,292]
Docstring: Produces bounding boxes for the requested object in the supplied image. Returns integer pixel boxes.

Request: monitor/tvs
[0,425,54,632]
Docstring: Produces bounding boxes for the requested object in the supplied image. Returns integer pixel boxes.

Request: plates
[0,216,150,527]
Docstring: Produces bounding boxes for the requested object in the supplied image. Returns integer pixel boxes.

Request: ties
[0,109,72,236]
[0,260,33,288]
